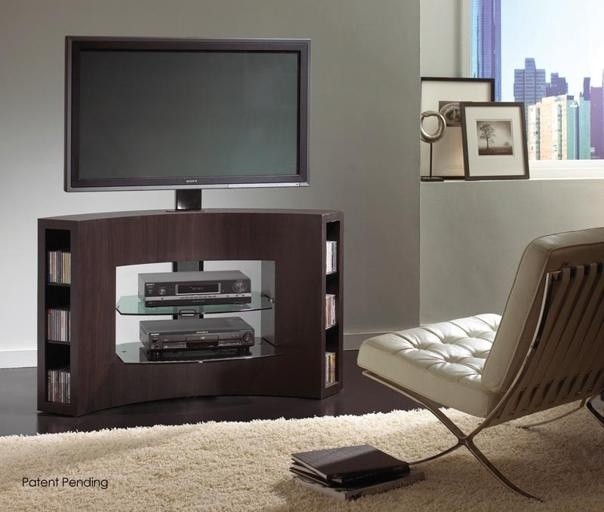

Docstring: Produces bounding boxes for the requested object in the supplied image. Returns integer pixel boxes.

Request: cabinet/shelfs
[37,209,344,417]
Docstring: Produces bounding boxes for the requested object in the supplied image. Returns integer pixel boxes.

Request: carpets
[0,394,604,511]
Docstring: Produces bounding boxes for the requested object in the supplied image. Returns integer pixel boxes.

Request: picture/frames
[419,77,529,181]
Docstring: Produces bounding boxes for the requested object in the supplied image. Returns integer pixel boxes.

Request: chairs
[357,227,604,502]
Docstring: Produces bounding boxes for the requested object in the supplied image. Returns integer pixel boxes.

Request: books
[325,241,337,385]
[290,445,425,501]
[47,251,71,404]
